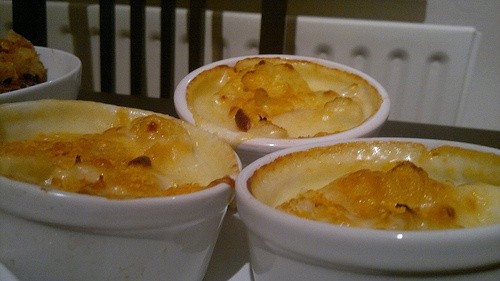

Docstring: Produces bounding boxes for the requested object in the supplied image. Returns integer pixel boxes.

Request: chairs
[97,1,287,102]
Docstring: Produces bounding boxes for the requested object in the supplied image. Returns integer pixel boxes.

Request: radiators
[45,0,484,127]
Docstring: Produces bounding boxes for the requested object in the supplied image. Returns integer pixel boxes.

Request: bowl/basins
[174,54,392,162]
[0,100,242,281]
[235,138,500,281]
[0,47,83,102]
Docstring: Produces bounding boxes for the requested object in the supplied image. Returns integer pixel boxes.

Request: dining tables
[2,90,500,280]
